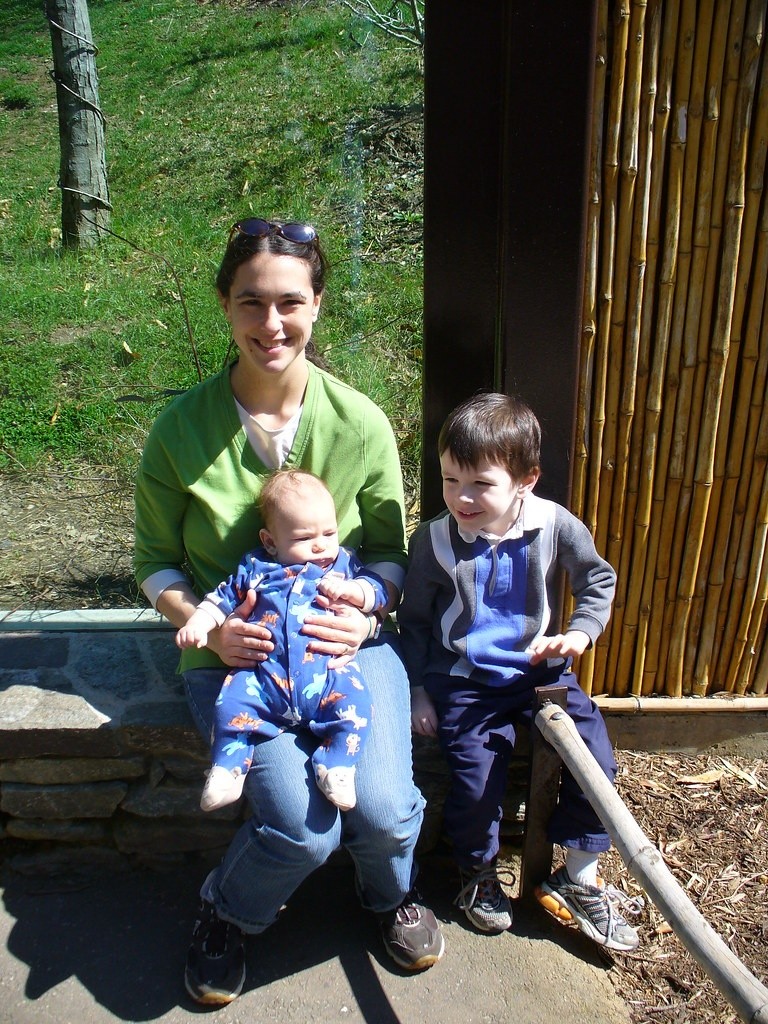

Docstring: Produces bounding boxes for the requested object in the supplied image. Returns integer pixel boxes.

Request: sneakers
[183,898,247,1007]
[453,865,516,934]
[373,886,445,973]
[531,862,641,951]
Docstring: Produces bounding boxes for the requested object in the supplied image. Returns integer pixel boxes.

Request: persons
[134,217,445,1004]
[175,465,389,812]
[397,394,643,950]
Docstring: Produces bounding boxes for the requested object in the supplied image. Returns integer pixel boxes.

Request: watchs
[372,610,384,641]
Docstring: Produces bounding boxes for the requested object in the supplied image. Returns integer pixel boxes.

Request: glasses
[229,217,321,246]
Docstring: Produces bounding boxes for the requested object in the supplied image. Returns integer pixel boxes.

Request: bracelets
[366,613,372,640]
